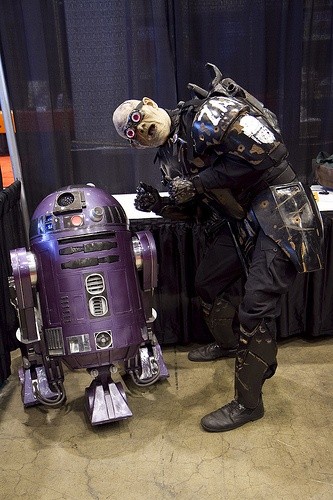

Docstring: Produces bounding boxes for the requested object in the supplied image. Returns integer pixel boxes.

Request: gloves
[167,176,203,202]
[132,181,164,214]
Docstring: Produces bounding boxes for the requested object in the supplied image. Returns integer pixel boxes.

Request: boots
[188,296,242,360]
[199,322,276,431]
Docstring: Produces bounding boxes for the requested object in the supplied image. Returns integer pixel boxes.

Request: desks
[113,184,332,345]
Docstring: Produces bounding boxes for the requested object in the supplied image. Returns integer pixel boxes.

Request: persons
[112,97,316,432]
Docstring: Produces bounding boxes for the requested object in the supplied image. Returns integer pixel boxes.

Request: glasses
[124,101,145,144]
